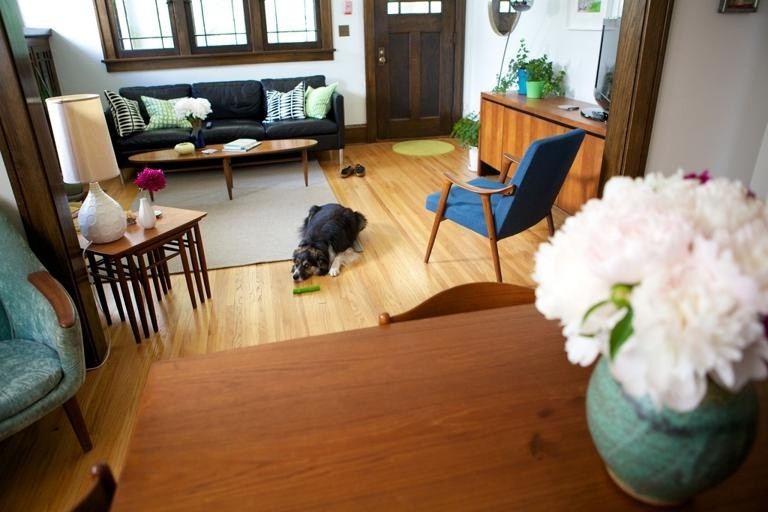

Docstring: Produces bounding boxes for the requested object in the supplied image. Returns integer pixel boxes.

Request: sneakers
[340,164,365,178]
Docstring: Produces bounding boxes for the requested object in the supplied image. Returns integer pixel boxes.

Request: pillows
[103,90,146,137]
[261,79,308,124]
[138,94,193,132]
[302,81,339,120]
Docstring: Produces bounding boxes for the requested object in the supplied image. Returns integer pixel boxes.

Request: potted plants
[492,39,536,96]
[448,110,480,173]
[526,53,570,101]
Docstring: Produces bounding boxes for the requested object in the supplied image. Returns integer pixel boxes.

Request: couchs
[102,74,347,186]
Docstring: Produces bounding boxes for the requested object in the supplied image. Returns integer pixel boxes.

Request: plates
[133,210,164,218]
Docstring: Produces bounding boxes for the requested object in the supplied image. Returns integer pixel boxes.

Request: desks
[75,204,211,346]
[477,91,607,217]
[109,300,768,511]
[128,137,319,199]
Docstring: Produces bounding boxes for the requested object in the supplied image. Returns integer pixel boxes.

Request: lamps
[43,91,128,244]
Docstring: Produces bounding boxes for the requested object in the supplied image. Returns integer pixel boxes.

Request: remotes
[205,122,212,129]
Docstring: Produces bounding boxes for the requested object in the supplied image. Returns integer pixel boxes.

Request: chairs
[1,205,87,455]
[424,126,588,284]
[375,279,535,324]
[68,462,117,510]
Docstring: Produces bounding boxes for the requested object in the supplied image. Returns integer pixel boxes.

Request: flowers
[134,166,168,198]
[528,166,768,412]
[170,94,214,121]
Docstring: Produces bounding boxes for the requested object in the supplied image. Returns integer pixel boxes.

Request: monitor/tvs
[580,18,620,122]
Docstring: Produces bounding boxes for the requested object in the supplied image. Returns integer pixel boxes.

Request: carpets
[391,139,455,157]
[79,158,364,284]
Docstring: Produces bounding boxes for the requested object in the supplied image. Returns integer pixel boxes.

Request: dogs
[291,203,368,282]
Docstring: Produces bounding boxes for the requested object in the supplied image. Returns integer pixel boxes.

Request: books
[222,142,262,153]
[224,139,257,149]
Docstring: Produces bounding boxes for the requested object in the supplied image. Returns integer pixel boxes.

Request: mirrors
[487,0,522,38]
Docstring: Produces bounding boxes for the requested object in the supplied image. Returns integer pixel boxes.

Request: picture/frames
[717,0,761,14]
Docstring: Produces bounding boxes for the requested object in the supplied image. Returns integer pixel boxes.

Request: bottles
[138,198,156,229]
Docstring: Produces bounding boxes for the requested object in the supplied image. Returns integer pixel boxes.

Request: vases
[186,116,206,149]
[137,196,157,230]
[585,355,759,510]
